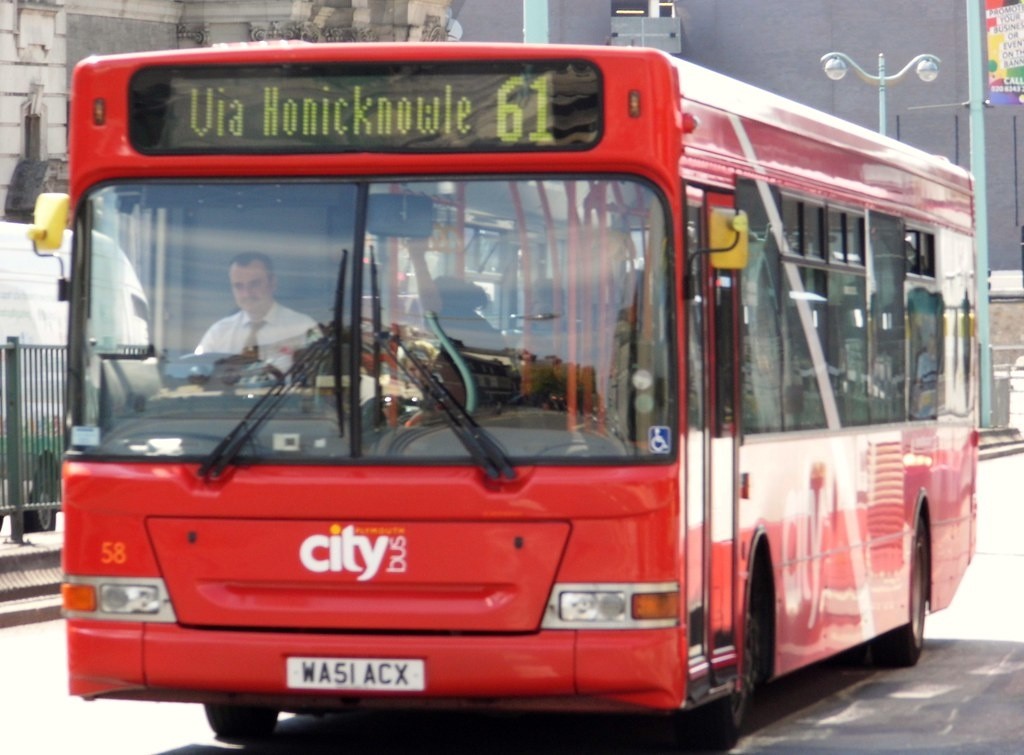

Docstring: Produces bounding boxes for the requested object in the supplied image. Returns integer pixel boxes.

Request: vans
[0,222,162,533]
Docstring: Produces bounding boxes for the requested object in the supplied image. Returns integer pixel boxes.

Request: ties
[242,321,265,358]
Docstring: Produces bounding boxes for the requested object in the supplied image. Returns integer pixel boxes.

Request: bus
[27,40,983,754]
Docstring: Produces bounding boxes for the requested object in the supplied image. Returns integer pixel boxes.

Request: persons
[396,341,438,389]
[421,274,505,353]
[194,253,331,386]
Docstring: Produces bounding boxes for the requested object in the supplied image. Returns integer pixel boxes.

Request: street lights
[819,51,945,141]
